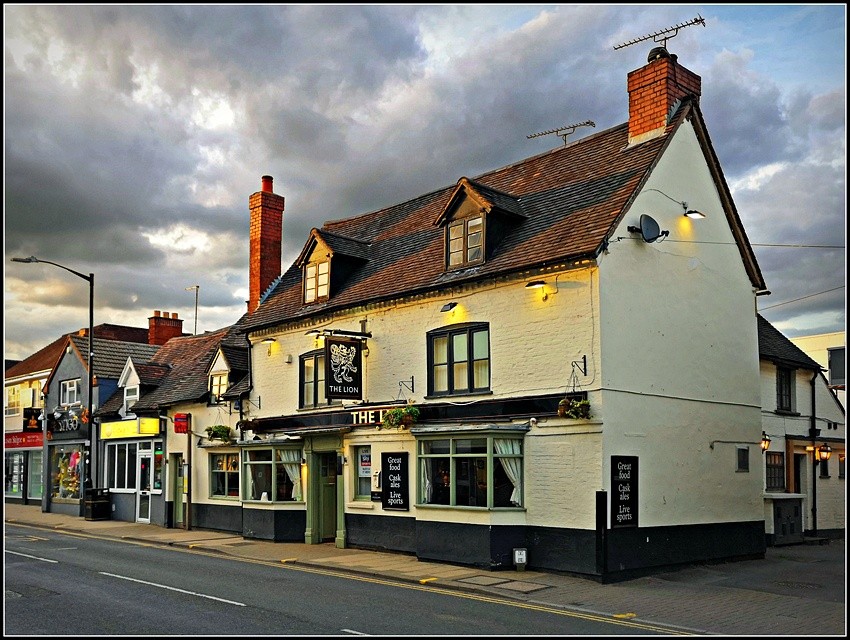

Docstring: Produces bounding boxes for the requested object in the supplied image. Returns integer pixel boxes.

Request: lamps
[37,408,47,420]
[301,458,306,465]
[815,442,832,464]
[524,279,545,289]
[441,303,457,313]
[762,430,771,453]
[305,330,320,337]
[261,338,276,344]
[684,210,706,219]
[53,406,62,419]
[341,455,347,464]
[69,403,76,417]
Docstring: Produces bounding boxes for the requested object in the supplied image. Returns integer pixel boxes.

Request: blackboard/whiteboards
[381,452,409,511]
[611,455,639,528]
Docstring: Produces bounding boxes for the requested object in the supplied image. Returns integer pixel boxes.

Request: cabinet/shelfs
[83,488,110,520]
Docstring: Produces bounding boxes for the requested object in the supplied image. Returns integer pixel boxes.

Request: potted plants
[382,407,419,427]
[206,425,230,442]
[559,399,589,416]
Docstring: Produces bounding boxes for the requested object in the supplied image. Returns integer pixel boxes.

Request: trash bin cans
[83,487,110,521]
